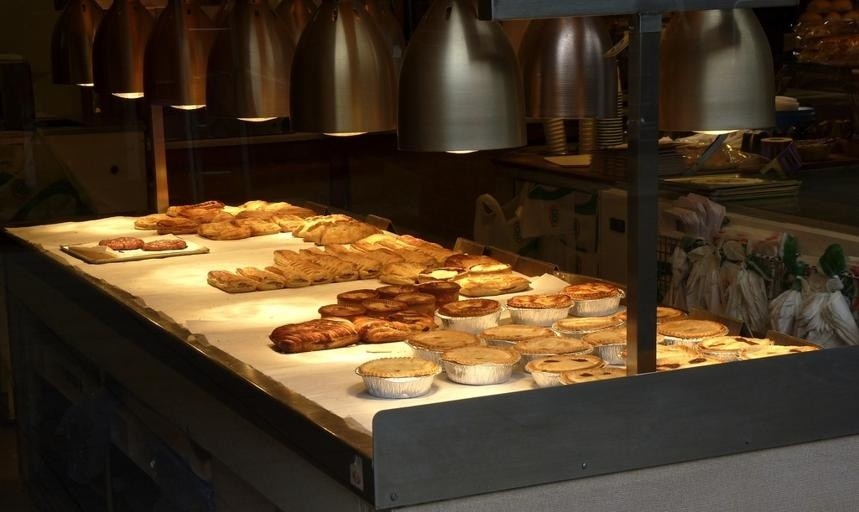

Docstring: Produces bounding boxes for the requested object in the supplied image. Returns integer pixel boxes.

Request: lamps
[289,1,397,138]
[150,0,209,111]
[203,2,290,124]
[655,9,776,136]
[396,2,529,156]
[520,16,618,119]
[51,0,104,88]
[91,0,152,99]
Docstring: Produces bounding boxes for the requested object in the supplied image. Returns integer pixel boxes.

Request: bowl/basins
[355,288,816,399]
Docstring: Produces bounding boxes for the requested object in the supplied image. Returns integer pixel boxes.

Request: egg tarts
[357,261,828,385]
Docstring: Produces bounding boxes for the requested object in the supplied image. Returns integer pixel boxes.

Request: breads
[99,198,497,353]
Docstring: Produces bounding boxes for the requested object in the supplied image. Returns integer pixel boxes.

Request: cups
[740,129,794,164]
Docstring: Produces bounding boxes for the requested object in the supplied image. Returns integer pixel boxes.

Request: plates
[591,90,697,177]
